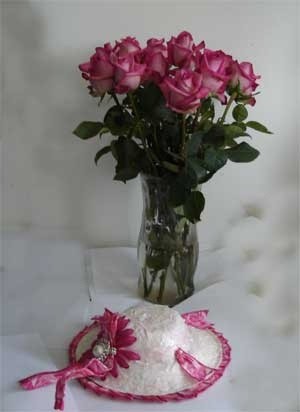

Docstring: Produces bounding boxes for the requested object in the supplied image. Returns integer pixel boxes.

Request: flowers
[73,30,275,225]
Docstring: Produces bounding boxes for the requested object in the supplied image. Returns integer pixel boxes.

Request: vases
[136,172,200,308]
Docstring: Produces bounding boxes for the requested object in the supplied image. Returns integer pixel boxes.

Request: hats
[18,302,231,408]
